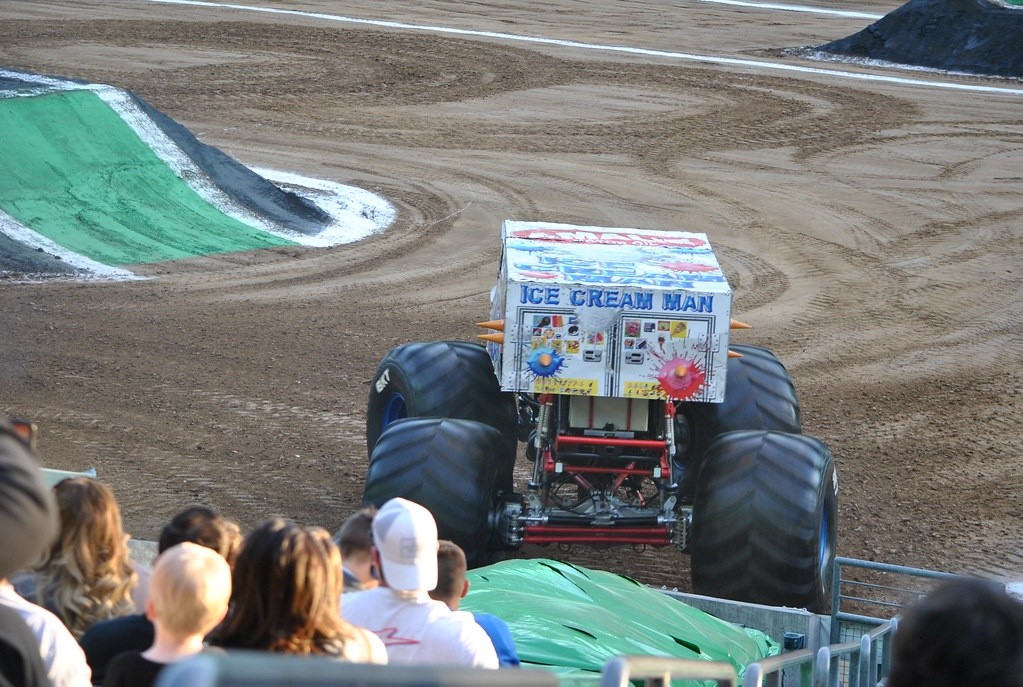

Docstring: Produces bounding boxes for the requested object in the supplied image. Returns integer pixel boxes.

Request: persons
[337,510,375,590]
[340,495,499,671]
[0,418,388,687]
[428,539,519,670]
[36,476,137,644]
[102,541,232,687]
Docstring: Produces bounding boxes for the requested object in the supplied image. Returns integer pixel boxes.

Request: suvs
[361,219,840,616]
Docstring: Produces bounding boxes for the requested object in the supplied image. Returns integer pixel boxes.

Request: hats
[371,497,438,592]
